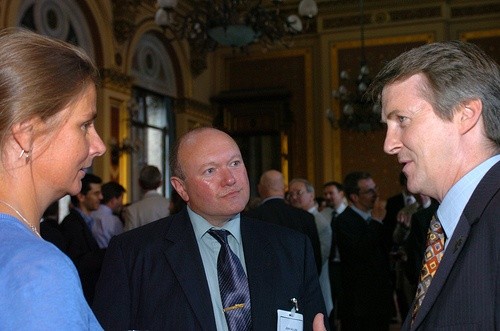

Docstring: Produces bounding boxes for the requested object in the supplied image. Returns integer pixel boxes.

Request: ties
[207,229,254,331]
[409,215,445,329]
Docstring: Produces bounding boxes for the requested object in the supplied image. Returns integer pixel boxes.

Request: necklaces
[0,200,45,241]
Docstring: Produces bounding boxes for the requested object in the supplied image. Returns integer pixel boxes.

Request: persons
[91,126,329,331]
[39,165,440,331]
[0,28,107,331]
[313,40,500,331]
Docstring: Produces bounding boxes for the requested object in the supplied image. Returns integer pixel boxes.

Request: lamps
[326,0,385,133]
[153,0,319,58]
